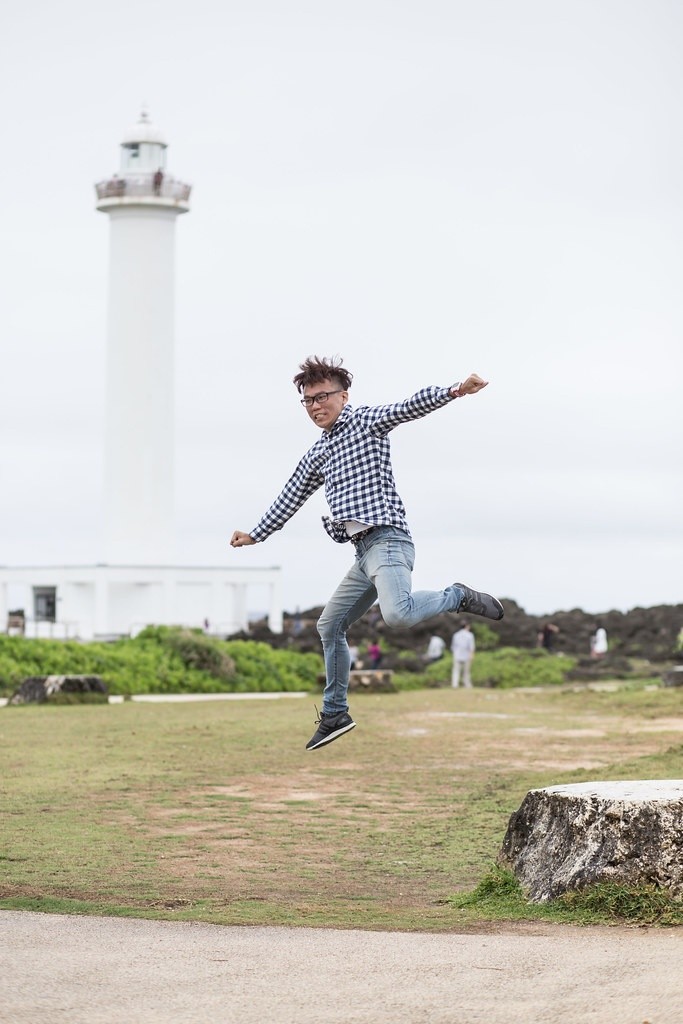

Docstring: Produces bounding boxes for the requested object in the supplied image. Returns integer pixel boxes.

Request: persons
[536,620,566,654]
[451,618,475,689]
[230,357,505,752]
[590,621,609,660]
[367,639,382,670]
[152,165,165,196]
[348,638,358,671]
[104,176,126,194]
[422,629,447,664]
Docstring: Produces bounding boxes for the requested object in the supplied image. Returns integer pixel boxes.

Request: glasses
[300,390,346,407]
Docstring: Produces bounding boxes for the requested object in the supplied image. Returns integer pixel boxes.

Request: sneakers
[451,582,506,622]
[306,711,357,751]
